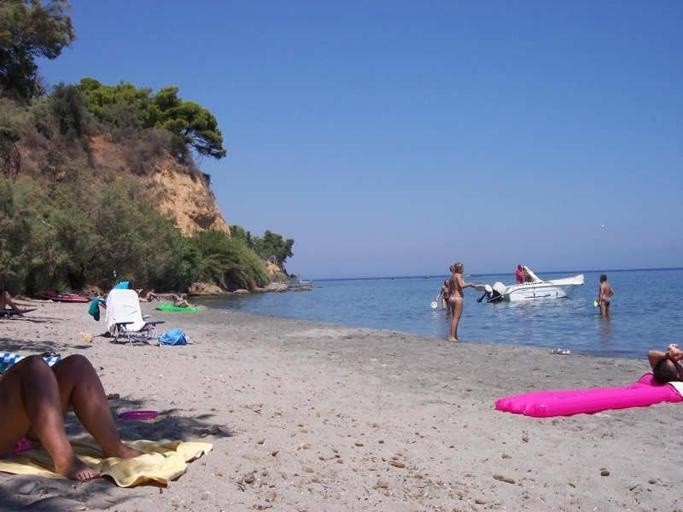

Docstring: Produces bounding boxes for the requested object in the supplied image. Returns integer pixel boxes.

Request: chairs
[105,289,164,348]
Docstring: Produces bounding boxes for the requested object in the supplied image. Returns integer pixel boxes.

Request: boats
[473,265,585,303]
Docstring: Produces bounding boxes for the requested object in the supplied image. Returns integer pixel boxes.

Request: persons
[0,280,24,316]
[646,342,682,383]
[595,274,615,319]
[134,287,198,309]
[0,351,145,482]
[513,264,531,286]
[435,278,452,311]
[446,261,478,344]
